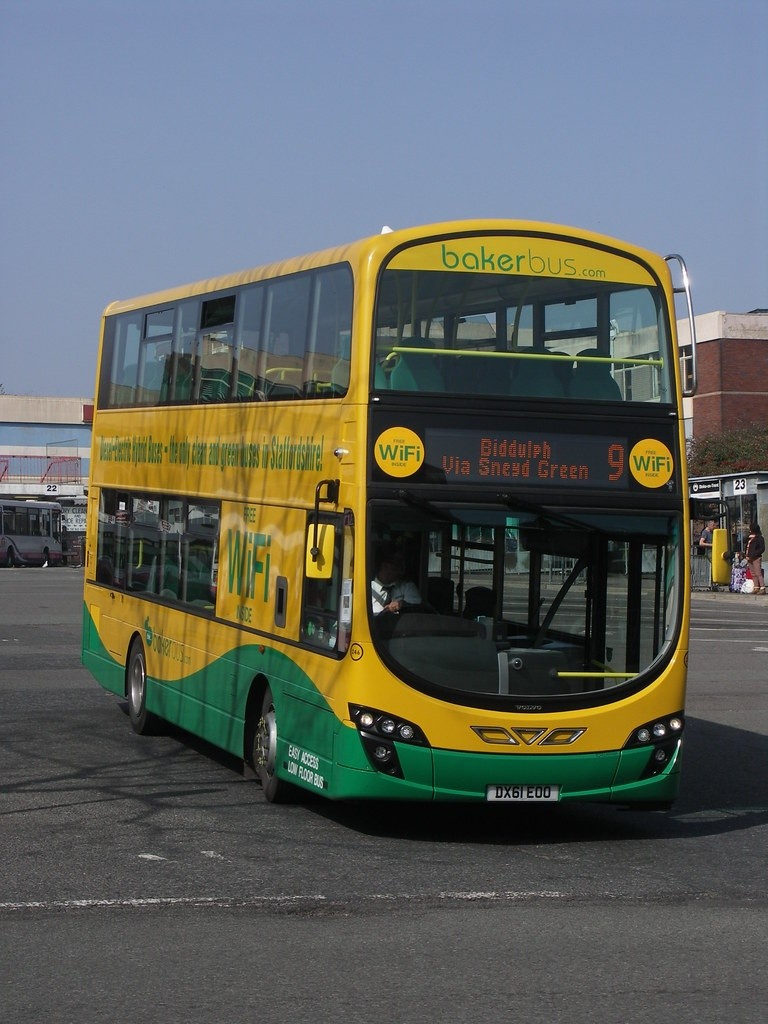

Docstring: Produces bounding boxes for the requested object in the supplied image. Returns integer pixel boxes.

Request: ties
[382,586,392,606]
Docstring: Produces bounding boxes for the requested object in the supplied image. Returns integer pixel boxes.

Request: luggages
[730,564,747,593]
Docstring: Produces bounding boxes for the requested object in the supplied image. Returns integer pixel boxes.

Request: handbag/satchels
[741,578,755,594]
[697,545,705,555]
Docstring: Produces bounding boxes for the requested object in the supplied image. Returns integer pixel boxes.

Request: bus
[0,500,66,568]
[80,218,734,805]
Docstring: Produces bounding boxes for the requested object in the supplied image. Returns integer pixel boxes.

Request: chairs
[183,556,212,600]
[477,351,515,394]
[148,552,179,596]
[509,348,564,396]
[426,576,454,614]
[452,354,488,394]
[131,523,160,566]
[568,349,621,399]
[463,586,495,620]
[391,337,446,392]
[117,348,350,403]
[101,523,127,569]
[331,334,389,395]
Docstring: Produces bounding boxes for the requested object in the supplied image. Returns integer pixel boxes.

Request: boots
[750,587,759,594]
[756,586,766,595]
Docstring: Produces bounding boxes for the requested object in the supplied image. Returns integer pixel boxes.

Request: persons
[62,526,68,535]
[371,541,422,614]
[744,523,766,595]
[699,520,719,558]
